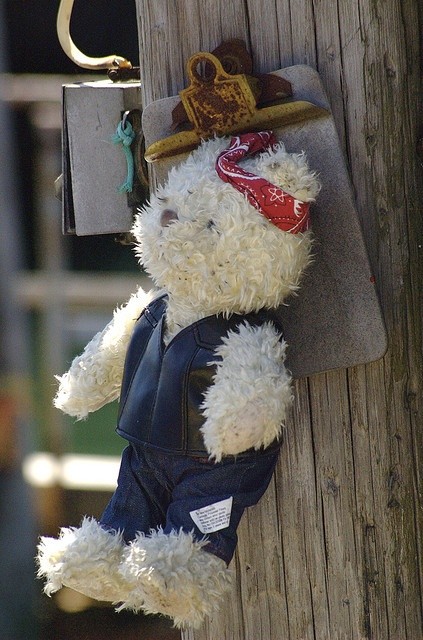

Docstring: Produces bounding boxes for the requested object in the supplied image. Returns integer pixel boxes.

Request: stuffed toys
[33,134,322,630]
